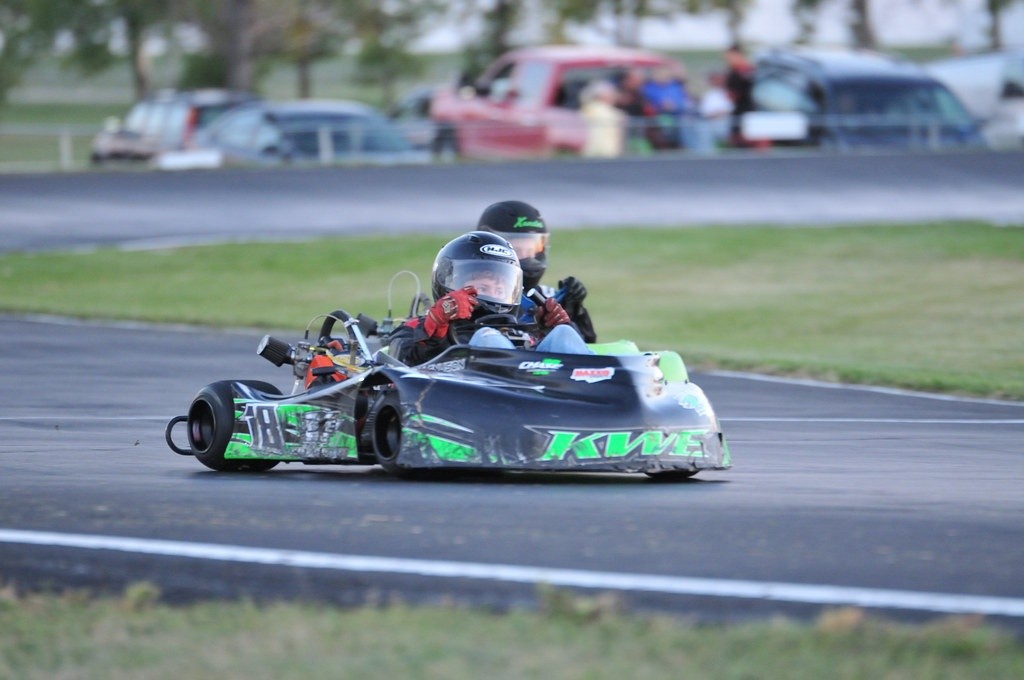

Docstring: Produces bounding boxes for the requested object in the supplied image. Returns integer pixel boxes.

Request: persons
[389,194,597,372]
[576,41,758,152]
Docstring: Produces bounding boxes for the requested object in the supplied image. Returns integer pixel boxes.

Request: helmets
[476,201,550,292]
[432,231,523,330]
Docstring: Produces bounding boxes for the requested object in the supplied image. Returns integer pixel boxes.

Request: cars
[742,46,992,151]
[90,90,434,170]
[425,45,690,162]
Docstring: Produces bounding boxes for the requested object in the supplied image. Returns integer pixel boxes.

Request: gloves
[559,277,587,319]
[536,296,570,340]
[424,286,478,339]
